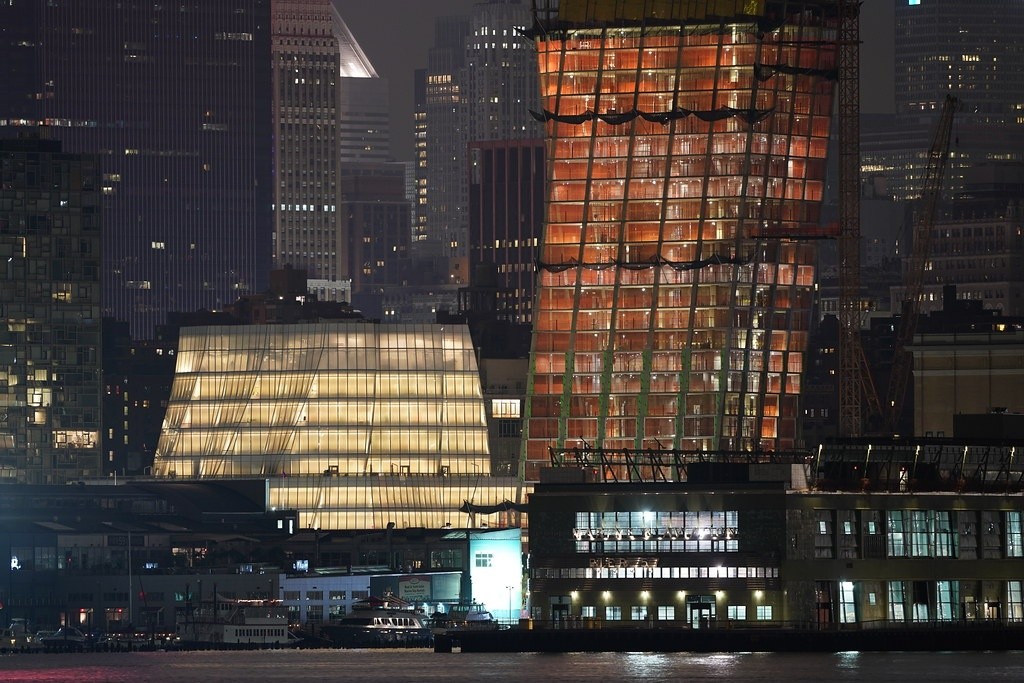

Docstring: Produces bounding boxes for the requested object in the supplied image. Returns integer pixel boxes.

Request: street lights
[506,587,514,628]
[64,557,72,643]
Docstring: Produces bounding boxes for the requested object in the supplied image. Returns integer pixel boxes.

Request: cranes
[876,92,965,439]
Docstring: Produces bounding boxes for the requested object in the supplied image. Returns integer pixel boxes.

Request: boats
[424,599,498,640]
[160,582,306,650]
[320,593,430,648]
